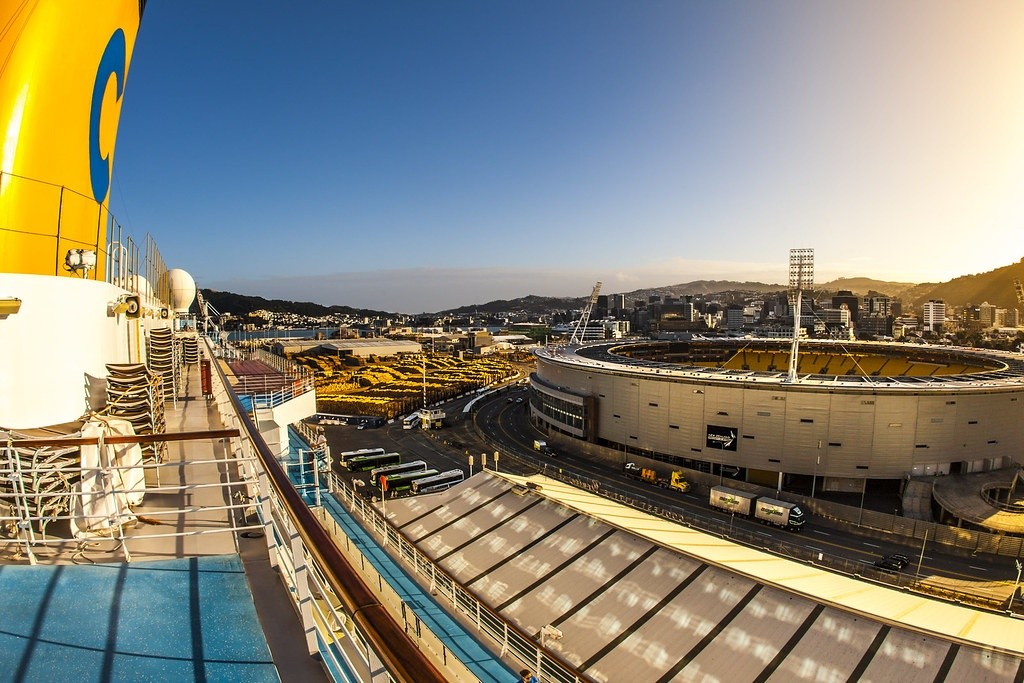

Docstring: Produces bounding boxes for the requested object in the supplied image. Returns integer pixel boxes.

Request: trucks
[534,439,546,452]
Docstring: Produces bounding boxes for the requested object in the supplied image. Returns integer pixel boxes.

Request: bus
[379,469,440,498]
[403,409,424,430]
[411,469,465,496]
[340,447,386,466]
[347,453,401,473]
[371,461,427,491]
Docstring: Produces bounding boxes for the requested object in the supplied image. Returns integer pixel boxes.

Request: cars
[545,449,559,457]
[505,397,523,405]
[872,552,908,574]
[357,422,367,430]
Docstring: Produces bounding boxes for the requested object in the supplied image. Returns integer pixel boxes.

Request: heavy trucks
[709,485,807,534]
[625,461,690,493]
